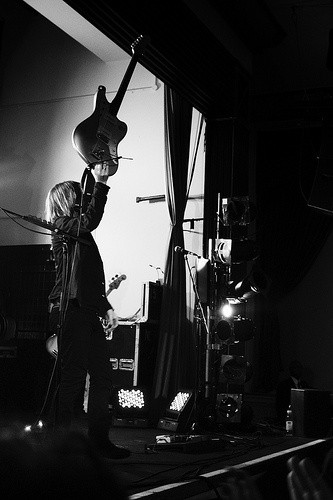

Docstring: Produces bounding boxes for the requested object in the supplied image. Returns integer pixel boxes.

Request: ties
[298,381,303,389]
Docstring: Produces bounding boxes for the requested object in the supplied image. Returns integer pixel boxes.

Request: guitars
[45,274,127,358]
[72,34,147,176]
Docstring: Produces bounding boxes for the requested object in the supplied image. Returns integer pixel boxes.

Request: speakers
[290,389,333,437]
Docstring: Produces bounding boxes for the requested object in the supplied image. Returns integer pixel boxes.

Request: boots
[88,424,133,464]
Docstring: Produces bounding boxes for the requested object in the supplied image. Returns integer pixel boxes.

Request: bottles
[287,410,293,432]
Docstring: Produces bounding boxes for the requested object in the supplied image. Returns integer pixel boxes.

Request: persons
[42,164,130,467]
[286,456,333,500]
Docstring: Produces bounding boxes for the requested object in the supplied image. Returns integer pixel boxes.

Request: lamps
[222,276,262,305]
[200,349,247,385]
[216,198,239,226]
[209,239,232,266]
[209,316,241,344]
[216,394,242,423]
[160,390,197,422]
[116,386,147,420]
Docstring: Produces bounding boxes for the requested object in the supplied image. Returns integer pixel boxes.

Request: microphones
[175,246,199,257]
